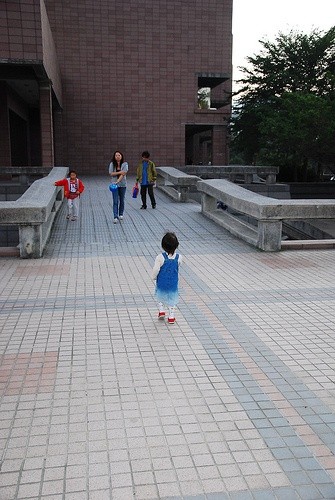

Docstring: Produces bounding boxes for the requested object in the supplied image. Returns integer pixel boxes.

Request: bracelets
[116,180,119,185]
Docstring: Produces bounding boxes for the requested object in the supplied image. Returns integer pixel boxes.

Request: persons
[136,151,158,210]
[109,152,129,224]
[53,167,84,220]
[198,161,212,166]
[149,233,182,324]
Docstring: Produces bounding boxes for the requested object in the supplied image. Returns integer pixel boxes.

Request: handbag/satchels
[132,180,139,198]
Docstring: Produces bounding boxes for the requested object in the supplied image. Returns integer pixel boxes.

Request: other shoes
[120,215,123,219]
[168,317,175,323]
[113,218,117,224]
[152,203,156,209]
[140,205,147,209]
[158,312,165,317]
